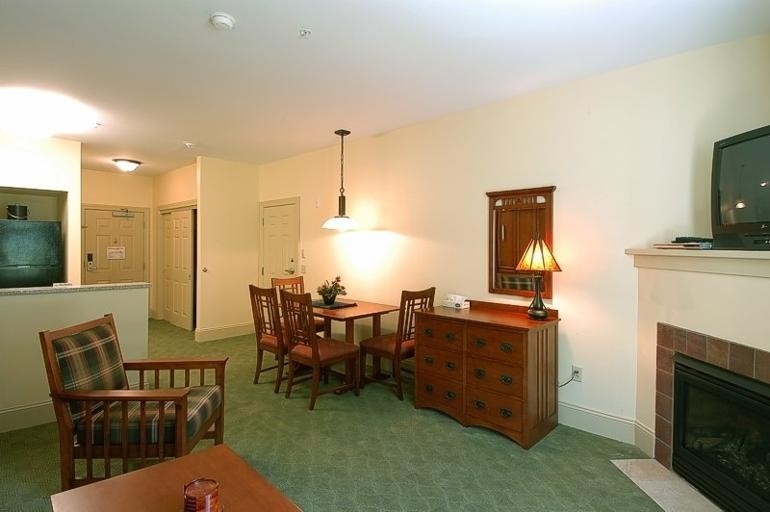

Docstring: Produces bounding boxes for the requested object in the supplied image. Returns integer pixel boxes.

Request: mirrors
[486,185,557,300]
[495,209,545,292]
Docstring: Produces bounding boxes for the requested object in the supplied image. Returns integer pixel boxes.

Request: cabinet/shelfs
[412,300,562,451]
[498,209,545,272]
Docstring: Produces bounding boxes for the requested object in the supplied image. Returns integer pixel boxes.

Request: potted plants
[317,276,347,305]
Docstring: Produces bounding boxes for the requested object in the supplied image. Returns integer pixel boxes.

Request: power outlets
[572,365,583,382]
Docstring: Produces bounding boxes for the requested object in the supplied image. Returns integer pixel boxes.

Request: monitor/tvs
[709,125,770,251]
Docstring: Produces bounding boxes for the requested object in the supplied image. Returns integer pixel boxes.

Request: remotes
[676,236,713,243]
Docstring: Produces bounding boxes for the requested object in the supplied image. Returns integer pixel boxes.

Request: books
[654,241,711,249]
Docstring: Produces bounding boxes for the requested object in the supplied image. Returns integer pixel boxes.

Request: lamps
[113,159,143,172]
[322,130,358,232]
[516,238,562,319]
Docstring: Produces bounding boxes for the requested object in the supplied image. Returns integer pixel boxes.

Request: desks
[278,298,406,394]
[50,444,304,512]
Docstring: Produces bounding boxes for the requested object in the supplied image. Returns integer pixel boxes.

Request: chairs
[271,276,325,360]
[279,289,360,410]
[360,287,435,401]
[496,273,543,290]
[249,284,322,393]
[39,313,229,492]
